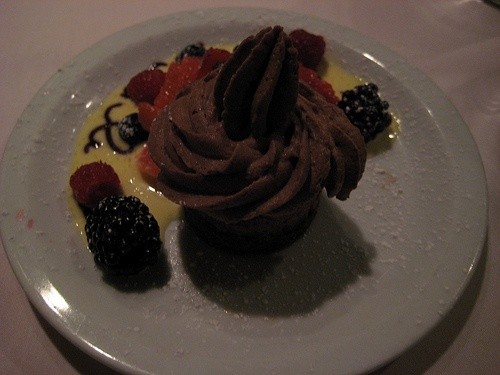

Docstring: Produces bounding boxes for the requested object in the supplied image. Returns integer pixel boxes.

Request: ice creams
[146,25,368,256]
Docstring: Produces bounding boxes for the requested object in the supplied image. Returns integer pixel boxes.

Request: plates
[1,8,487,375]
